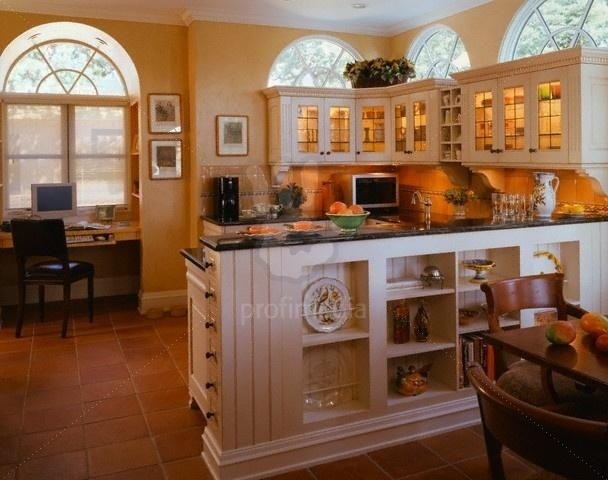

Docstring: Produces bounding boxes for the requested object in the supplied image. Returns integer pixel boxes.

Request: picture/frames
[147,94,183,180]
[216,116,249,156]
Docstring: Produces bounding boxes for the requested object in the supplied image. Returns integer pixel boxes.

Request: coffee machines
[213,176,239,222]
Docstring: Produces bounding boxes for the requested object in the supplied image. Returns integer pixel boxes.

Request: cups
[270,204,283,214]
[491,192,535,223]
[241,209,256,217]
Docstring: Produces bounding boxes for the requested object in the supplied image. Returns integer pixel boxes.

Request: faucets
[411,190,432,231]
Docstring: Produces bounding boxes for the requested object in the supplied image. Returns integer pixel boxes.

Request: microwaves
[338,173,404,209]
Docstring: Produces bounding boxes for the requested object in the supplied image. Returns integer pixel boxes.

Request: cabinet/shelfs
[448,55,531,168]
[356,87,391,165]
[530,46,608,170]
[391,78,457,164]
[262,86,355,166]
[438,84,462,163]
[300,239,580,424]
[204,246,222,447]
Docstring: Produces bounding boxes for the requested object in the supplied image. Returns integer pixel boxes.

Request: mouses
[96,236,106,241]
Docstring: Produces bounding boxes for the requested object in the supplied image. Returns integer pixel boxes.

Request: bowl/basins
[324,211,371,232]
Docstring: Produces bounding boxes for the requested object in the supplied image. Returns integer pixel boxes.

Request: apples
[330,202,363,215]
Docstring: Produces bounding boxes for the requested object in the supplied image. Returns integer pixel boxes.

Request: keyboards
[64,236,95,244]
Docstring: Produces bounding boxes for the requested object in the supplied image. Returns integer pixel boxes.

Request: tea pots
[529,171,559,219]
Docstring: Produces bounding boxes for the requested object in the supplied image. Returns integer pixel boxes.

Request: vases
[352,78,407,88]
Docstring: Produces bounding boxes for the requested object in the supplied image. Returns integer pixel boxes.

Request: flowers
[342,55,417,84]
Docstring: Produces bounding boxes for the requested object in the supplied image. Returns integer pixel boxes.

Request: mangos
[582,313,608,336]
[545,320,576,345]
[595,333,608,353]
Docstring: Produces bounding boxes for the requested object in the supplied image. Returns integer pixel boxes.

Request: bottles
[394,301,429,343]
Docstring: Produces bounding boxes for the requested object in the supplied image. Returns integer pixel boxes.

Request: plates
[235,227,285,241]
[238,214,256,221]
[458,308,481,324]
[481,303,510,317]
[283,224,326,235]
[303,278,351,333]
[557,210,586,220]
[302,347,349,412]
[462,259,496,271]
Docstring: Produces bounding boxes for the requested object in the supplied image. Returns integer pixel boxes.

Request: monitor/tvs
[31,182,78,219]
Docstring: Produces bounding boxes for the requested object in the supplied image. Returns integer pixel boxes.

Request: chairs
[467,273,607,479]
[11,218,94,337]
